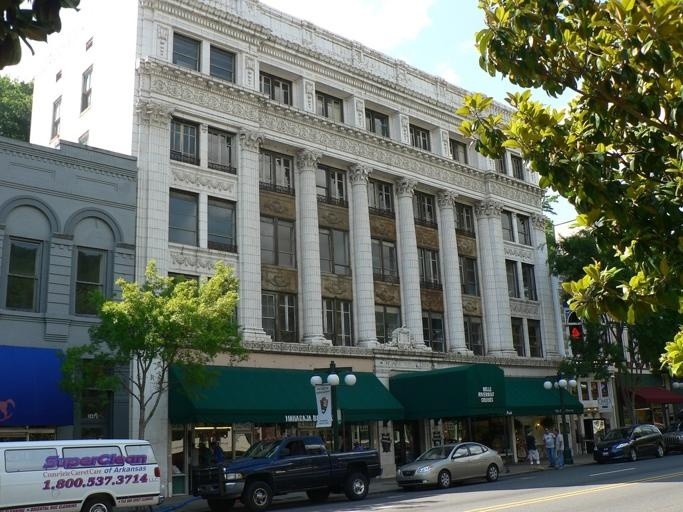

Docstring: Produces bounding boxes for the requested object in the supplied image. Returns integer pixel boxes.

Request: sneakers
[531,462,534,465]
[537,461,540,464]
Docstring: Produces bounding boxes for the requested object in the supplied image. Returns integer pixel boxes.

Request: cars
[663,419,683,453]
[397,443,504,490]
[593,423,667,463]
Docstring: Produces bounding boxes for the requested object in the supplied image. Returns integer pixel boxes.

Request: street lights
[543,370,577,465]
[311,360,357,455]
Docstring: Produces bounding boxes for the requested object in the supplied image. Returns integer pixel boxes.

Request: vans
[0,438,162,512]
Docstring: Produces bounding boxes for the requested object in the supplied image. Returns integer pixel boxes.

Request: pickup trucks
[194,437,381,510]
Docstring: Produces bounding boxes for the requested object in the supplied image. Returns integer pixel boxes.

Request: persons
[352,442,364,451]
[190,443,199,497]
[542,427,556,467]
[211,441,225,465]
[197,443,214,467]
[553,429,565,469]
[526,428,540,464]
